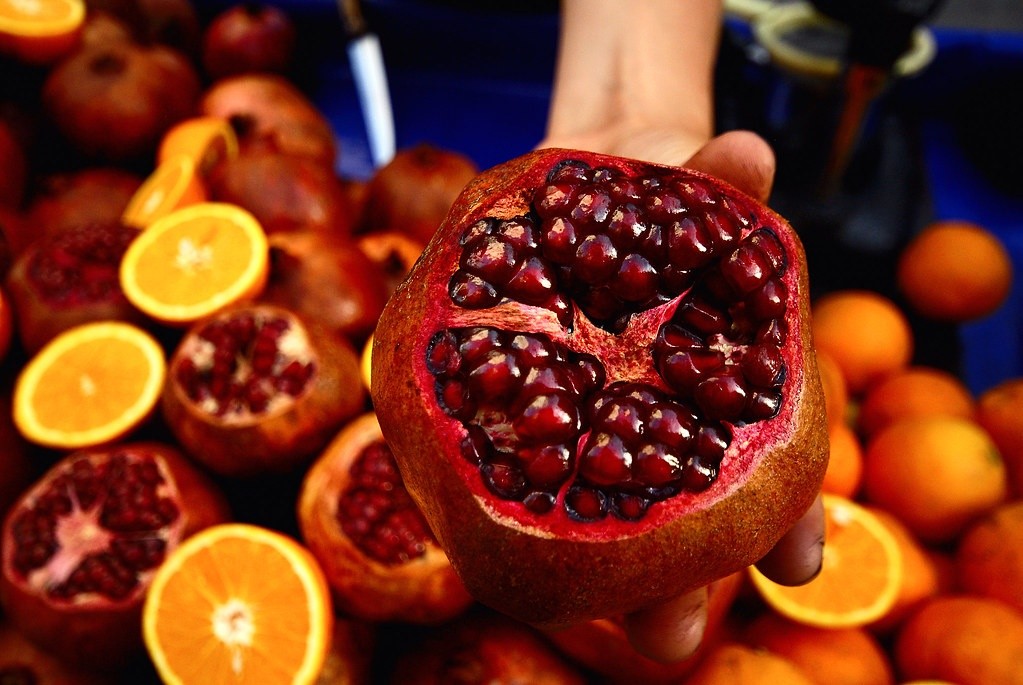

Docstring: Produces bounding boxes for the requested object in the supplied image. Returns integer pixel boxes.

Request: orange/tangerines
[459,225,1023,685]
[123,203,267,314]
[0,2,85,58]
[143,524,330,685]
[14,317,167,443]
[128,119,239,226]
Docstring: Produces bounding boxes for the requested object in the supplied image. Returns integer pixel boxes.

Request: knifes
[337,0,398,169]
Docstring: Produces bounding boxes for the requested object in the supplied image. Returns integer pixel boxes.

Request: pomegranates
[2,444,227,685]
[160,304,467,616]
[204,8,463,317]
[372,146,827,616]
[0,46,186,315]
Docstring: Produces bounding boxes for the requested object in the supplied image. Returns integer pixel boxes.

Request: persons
[357,2,833,663]
